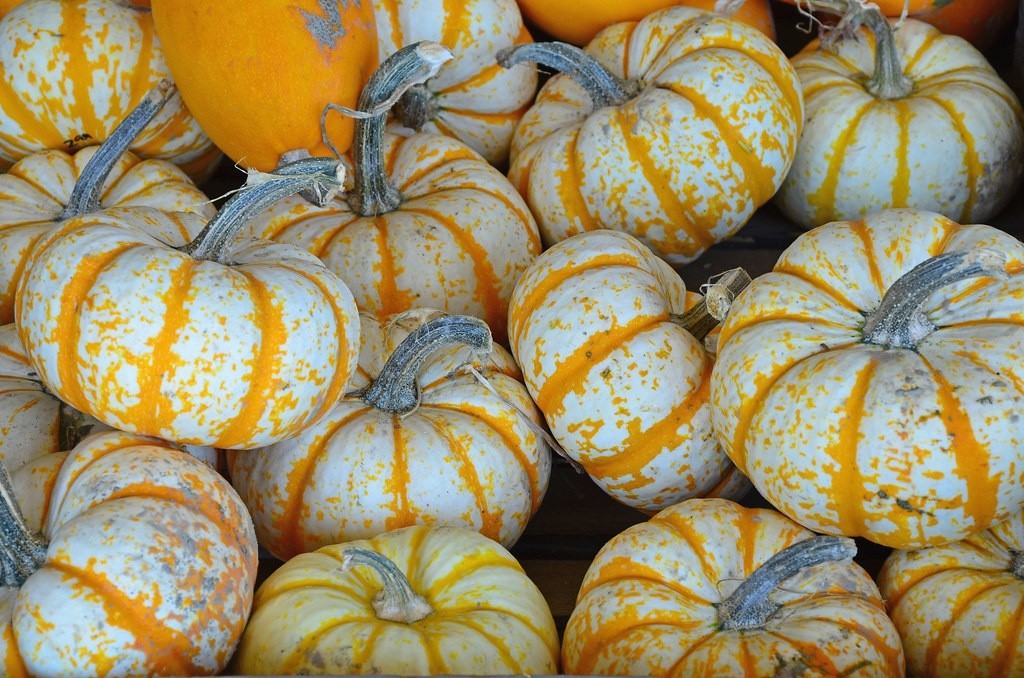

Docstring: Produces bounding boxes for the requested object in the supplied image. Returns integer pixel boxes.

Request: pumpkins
[1,0,1021,678]
